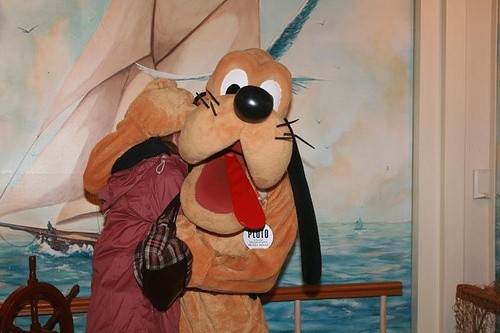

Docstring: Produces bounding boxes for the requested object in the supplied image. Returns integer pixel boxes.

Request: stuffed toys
[170,47,321,333]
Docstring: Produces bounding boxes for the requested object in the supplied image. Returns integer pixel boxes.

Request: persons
[82,78,191,332]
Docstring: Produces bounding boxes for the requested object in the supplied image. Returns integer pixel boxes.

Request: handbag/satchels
[133,192,193,311]
